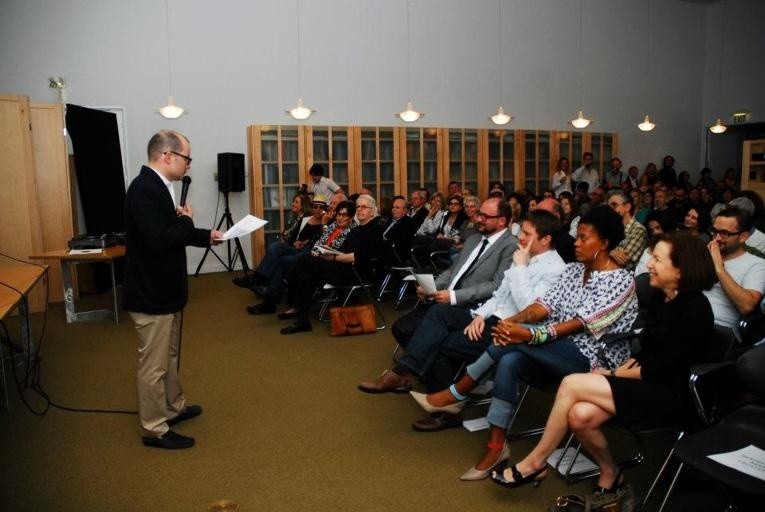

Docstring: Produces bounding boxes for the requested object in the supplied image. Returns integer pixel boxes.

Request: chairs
[281,203,765,512]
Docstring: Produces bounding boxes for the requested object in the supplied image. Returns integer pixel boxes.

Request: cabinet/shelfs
[248,123,620,272]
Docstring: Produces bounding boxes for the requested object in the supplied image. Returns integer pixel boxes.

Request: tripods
[195,192,249,277]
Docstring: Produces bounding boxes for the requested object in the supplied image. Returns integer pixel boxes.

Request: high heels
[594,466,626,497]
[490,462,552,490]
[408,390,471,418]
[458,439,512,484]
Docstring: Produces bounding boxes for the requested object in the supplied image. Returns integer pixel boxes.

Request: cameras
[585,164,591,169]
[613,166,618,175]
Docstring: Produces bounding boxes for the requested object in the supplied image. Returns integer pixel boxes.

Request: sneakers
[277,307,304,322]
[230,273,260,295]
[245,300,275,316]
[278,320,314,335]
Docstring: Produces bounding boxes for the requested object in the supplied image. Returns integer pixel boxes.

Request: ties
[451,238,489,290]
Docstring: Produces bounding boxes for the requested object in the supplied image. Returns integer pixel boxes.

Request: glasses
[710,226,741,242]
[309,198,508,223]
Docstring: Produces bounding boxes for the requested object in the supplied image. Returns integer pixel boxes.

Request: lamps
[631,2,657,133]
[283,0,318,120]
[708,2,728,135]
[567,2,593,130]
[393,0,425,123]
[156,2,184,119]
[485,0,517,127]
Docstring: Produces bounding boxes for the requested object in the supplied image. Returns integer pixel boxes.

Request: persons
[122,131,223,451]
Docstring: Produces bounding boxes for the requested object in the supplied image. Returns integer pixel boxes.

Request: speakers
[218,153,245,192]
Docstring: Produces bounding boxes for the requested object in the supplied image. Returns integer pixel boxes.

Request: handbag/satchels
[549,484,637,512]
[327,304,380,338]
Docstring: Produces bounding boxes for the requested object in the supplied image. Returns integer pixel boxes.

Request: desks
[26,244,128,327]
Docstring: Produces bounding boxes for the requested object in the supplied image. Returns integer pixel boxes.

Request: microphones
[179,176,191,217]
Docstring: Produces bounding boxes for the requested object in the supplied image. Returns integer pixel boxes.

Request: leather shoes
[356,368,415,395]
[141,428,196,451]
[412,411,465,433]
[165,404,203,426]
